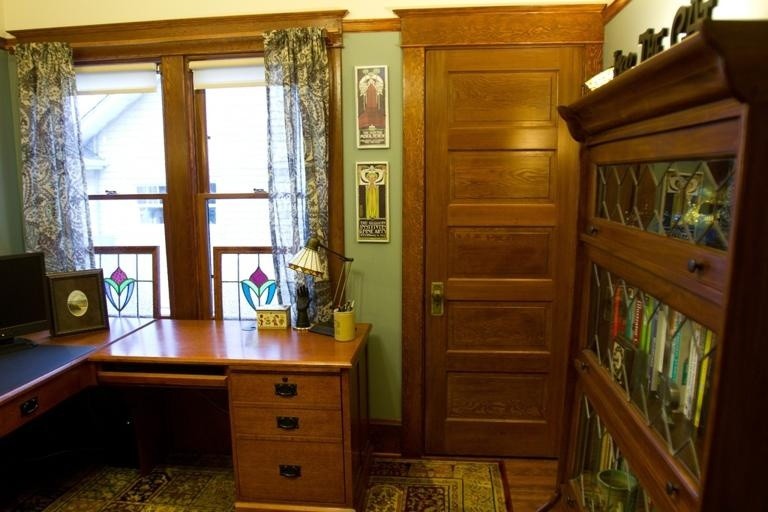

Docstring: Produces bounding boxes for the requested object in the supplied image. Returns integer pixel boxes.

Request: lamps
[288,238,357,336]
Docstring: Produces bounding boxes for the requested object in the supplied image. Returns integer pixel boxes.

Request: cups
[333,309,356,341]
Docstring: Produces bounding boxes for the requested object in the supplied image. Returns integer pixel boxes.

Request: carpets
[0,454,513,512]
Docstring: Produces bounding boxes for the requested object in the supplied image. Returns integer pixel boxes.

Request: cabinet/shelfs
[537,20,767,511]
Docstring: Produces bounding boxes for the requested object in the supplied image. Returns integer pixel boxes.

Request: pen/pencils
[338,299,355,312]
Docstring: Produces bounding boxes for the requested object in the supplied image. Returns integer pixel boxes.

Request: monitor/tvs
[0,253,51,350]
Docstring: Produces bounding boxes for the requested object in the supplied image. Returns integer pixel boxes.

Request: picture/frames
[43,268,109,337]
[355,162,389,243]
[355,65,389,149]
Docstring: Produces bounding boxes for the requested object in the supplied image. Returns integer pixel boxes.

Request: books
[606,281,711,445]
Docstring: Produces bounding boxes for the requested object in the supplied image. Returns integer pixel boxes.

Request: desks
[0,317,372,512]
